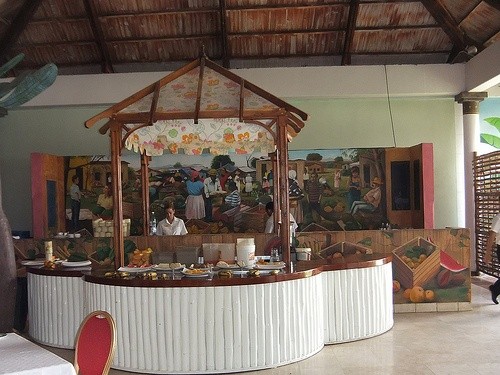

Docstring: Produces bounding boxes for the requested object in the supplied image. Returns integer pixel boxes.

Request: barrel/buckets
[236,239,255,266]
[202,243,218,265]
[297,251,311,261]
[219,243,236,264]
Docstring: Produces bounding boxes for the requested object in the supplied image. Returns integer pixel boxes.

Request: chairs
[72,310,118,375]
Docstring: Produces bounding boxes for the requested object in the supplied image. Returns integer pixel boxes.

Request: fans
[0,48,58,109]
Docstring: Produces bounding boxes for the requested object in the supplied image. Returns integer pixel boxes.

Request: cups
[55,232,80,237]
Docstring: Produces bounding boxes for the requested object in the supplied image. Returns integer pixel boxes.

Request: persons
[157,205,188,235]
[484,195,500,304]
[265,201,298,246]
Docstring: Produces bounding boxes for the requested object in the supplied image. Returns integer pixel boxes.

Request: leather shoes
[489,285,498,304]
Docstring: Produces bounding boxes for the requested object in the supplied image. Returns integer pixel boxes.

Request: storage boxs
[392,236,440,288]
[301,223,330,232]
[88,249,116,267]
[315,240,373,259]
[183,218,211,233]
[122,201,143,221]
[240,204,271,232]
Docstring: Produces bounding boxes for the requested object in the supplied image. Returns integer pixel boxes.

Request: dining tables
[0,331,78,375]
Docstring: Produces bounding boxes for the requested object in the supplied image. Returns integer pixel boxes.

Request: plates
[20,259,92,266]
[117,260,285,277]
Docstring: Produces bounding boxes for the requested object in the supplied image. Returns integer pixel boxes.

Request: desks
[26,252,395,375]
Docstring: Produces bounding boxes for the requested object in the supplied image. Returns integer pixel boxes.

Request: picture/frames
[59,147,386,231]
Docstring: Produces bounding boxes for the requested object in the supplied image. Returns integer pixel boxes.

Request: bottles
[271,248,279,262]
[150,211,157,236]
